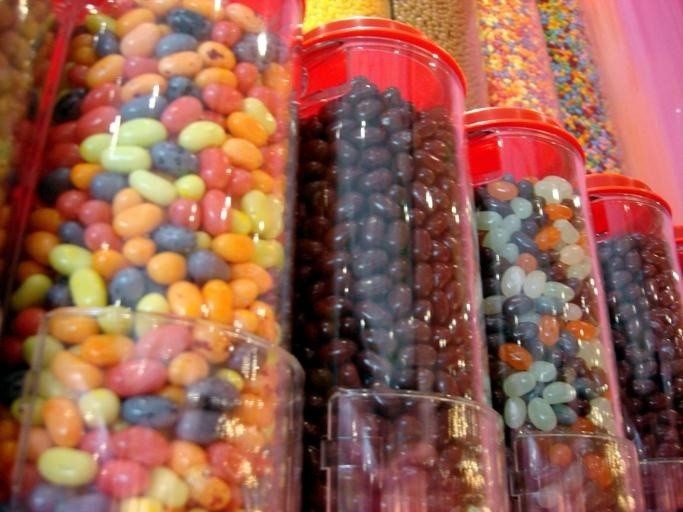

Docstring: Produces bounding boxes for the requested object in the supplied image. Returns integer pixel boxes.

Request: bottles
[481,0,564,130]
[286,22,505,512]
[3,0,308,511]
[392,0,485,115]
[536,0,631,180]
[462,108,642,512]
[581,176,683,512]
[306,0,397,42]
[0,0,84,336]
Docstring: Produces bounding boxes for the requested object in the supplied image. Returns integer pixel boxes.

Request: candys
[597,232,683,451]
[299,80,488,399]
[515,428,681,511]
[469,170,619,436]
[0,0,300,340]
[319,390,509,512]
[1,302,304,512]
